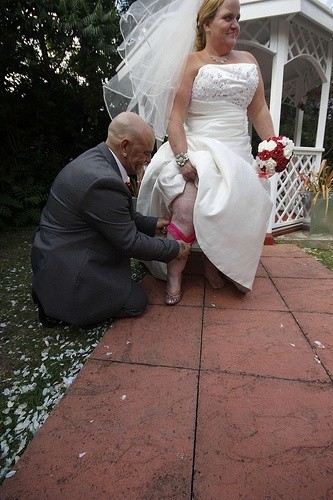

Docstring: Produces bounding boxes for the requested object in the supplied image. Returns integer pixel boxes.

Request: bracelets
[176,153,189,166]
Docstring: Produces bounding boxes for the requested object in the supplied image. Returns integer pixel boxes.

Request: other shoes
[32,291,55,328]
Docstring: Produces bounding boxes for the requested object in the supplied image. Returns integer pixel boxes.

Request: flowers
[256,135,294,179]
[175,152,187,167]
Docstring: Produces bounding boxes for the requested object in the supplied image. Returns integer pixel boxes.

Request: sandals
[164,286,182,304]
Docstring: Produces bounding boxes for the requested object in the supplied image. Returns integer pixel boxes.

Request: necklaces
[204,48,230,64]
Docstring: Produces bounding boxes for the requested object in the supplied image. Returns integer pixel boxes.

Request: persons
[30,110,191,327]
[102,0,277,305]
